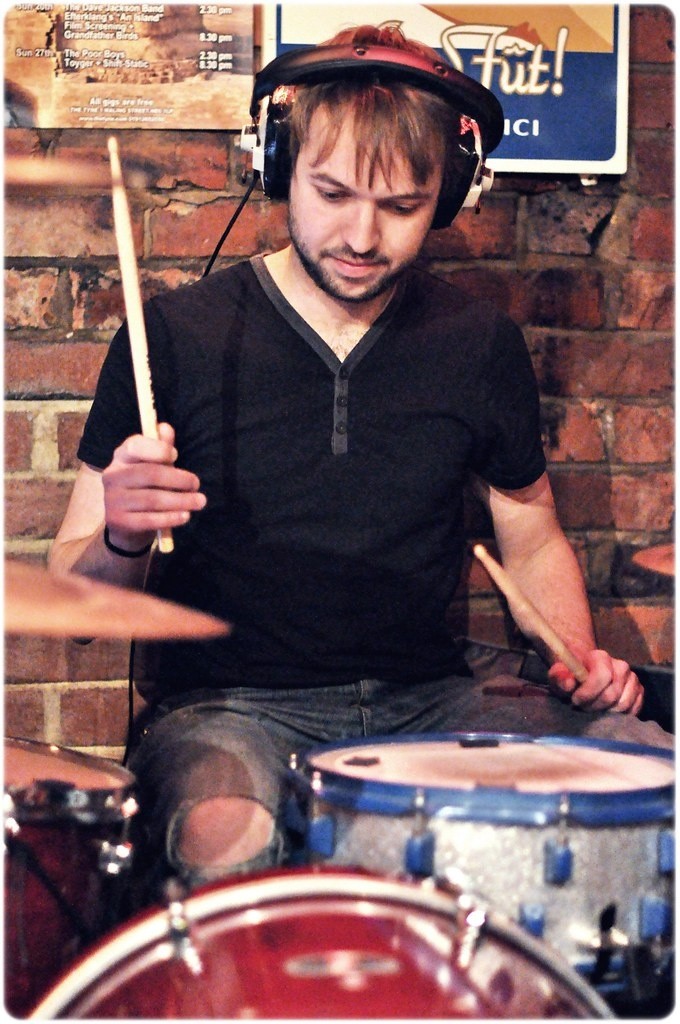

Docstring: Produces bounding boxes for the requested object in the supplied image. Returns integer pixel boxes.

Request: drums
[267,728,675,997]
[4,732,138,1019]
[22,863,622,1021]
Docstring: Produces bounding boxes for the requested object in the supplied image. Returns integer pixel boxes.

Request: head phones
[237,44,505,230]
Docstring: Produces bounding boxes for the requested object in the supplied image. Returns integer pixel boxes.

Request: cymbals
[631,542,676,579]
[3,556,231,640]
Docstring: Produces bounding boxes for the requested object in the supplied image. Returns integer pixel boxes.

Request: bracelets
[104,525,153,557]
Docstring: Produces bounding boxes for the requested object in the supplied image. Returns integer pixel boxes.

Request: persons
[49,25,646,921]
